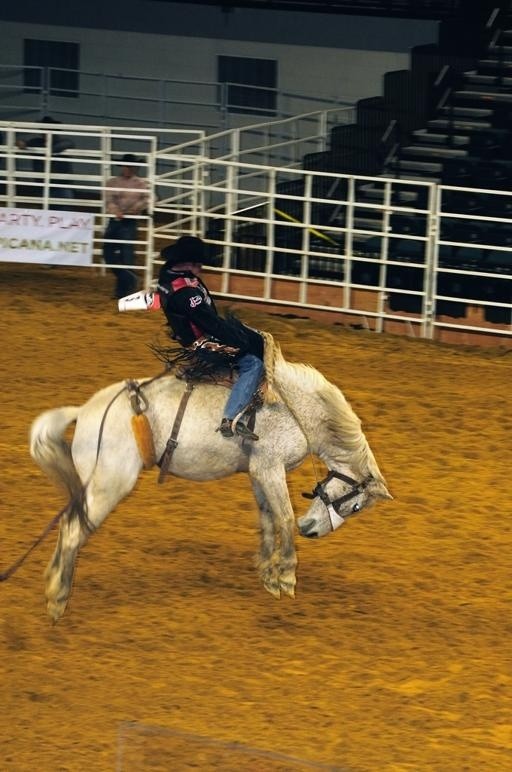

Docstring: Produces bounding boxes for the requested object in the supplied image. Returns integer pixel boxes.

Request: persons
[19,117,74,270]
[159,235,264,442]
[103,154,149,300]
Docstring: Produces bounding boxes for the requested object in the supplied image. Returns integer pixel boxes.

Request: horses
[29,341,394,627]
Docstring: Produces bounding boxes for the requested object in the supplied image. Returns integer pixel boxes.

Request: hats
[159,235,225,262]
[113,153,147,168]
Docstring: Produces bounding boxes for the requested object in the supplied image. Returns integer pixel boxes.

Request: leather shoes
[218,416,260,440]
